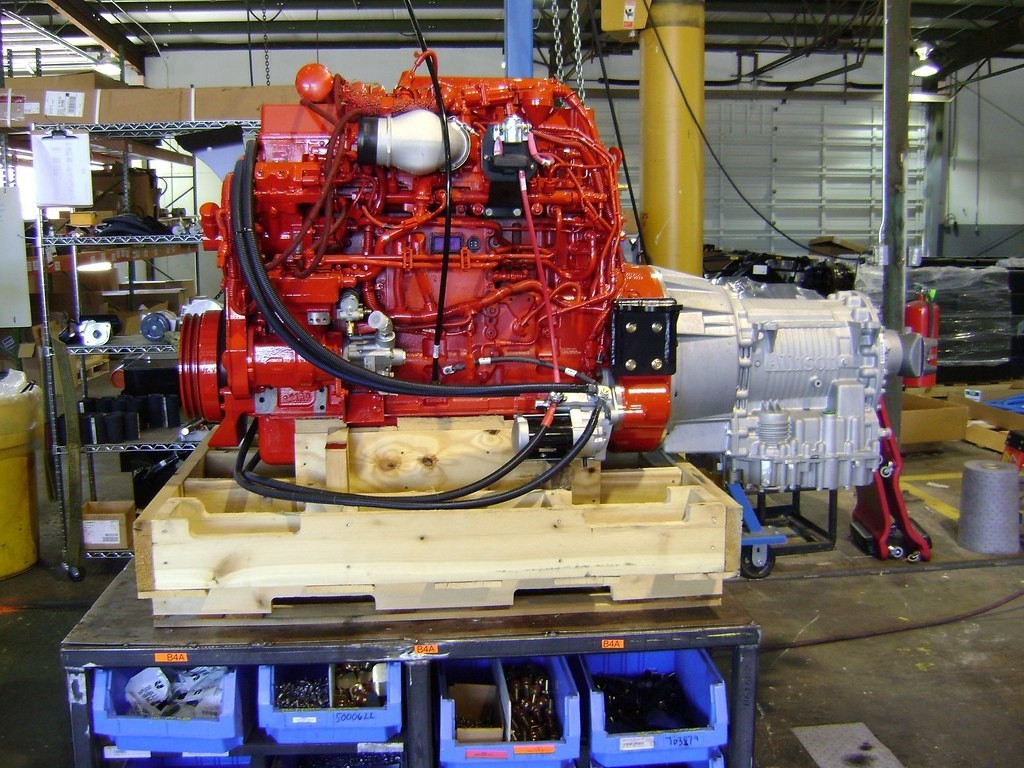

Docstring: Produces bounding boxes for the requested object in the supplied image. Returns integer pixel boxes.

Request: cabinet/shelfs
[30,117,262,584]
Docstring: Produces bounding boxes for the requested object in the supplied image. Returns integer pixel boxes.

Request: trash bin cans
[0,381,44,583]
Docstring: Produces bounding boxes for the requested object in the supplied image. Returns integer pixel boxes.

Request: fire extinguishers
[903,281,941,388]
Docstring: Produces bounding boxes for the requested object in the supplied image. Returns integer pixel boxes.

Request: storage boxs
[575,647,730,768]
[439,654,581,768]
[78,168,156,220]
[899,389,970,455]
[80,499,136,550]
[256,660,405,744]
[91,668,257,753]
